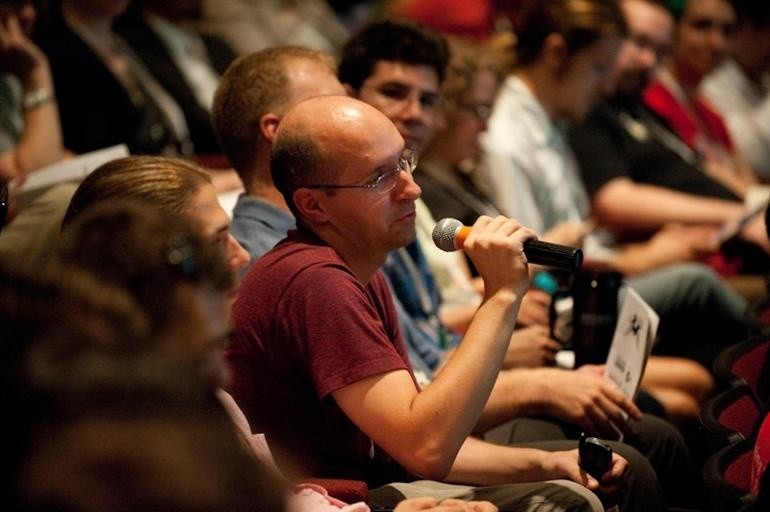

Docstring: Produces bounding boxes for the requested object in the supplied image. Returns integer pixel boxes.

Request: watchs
[20,89,55,109]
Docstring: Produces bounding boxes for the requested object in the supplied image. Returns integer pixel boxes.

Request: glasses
[300,141,419,195]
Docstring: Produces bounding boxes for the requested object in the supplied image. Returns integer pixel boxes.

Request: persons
[482,0,720,275]
[199,3,354,57]
[211,44,660,512]
[695,0,770,178]
[112,0,238,156]
[337,21,715,428]
[565,0,769,276]
[0,362,287,511]
[31,0,214,157]
[0,0,63,180]
[51,196,235,397]
[2,246,158,386]
[221,94,630,511]
[642,1,762,201]
[366,1,763,358]
[59,155,500,512]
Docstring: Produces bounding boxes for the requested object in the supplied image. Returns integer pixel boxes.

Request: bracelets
[19,88,57,115]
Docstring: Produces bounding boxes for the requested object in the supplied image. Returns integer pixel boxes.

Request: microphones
[432,215,584,272]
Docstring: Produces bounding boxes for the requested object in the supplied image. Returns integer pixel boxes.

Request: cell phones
[578,432,613,479]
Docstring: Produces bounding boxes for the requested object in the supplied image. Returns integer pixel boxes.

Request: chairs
[743,287,770,336]
[697,384,770,447]
[697,442,770,511]
[712,337,768,401]
